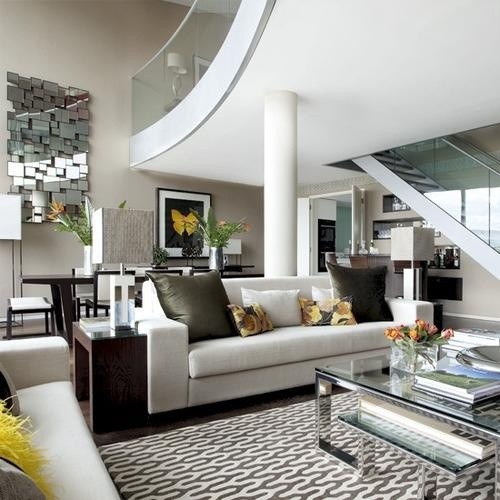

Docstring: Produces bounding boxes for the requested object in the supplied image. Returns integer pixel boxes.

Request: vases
[43,199,127,245]
[179,207,252,247]
[385,319,455,372]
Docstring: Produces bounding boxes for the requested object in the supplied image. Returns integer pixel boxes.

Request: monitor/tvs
[411,364,500,409]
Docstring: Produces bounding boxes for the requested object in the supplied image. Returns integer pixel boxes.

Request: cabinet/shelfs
[391,196,410,210]
[435,246,459,268]
[346,239,374,255]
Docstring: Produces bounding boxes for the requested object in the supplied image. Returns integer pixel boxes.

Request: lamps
[72,267,223,318]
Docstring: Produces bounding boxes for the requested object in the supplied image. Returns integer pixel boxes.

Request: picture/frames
[18,273,263,347]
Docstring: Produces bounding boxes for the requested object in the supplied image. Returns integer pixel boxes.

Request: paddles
[97,391,500,500]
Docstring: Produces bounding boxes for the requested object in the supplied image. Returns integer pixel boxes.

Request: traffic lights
[1,458,46,500]
[1,365,20,416]
[147,261,394,344]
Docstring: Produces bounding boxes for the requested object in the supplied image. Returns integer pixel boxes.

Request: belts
[376,223,410,238]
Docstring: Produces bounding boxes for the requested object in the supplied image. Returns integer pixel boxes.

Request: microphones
[6,296,55,339]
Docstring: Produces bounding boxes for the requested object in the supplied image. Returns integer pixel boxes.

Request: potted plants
[425,244,461,269]
[192,54,212,87]
[383,194,410,213]
[372,217,440,240]
[156,187,212,259]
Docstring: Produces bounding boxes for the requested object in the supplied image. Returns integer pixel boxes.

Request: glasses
[390,226,435,300]
[222,239,242,266]
[0,192,23,328]
[165,52,187,113]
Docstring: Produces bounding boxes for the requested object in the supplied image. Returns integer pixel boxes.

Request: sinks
[0,336,121,500]
[131,274,443,427]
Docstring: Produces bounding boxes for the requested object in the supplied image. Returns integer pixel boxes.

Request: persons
[392,196,401,211]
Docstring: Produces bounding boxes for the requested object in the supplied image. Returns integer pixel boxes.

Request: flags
[208,246,224,271]
[391,341,438,375]
[83,245,98,276]
